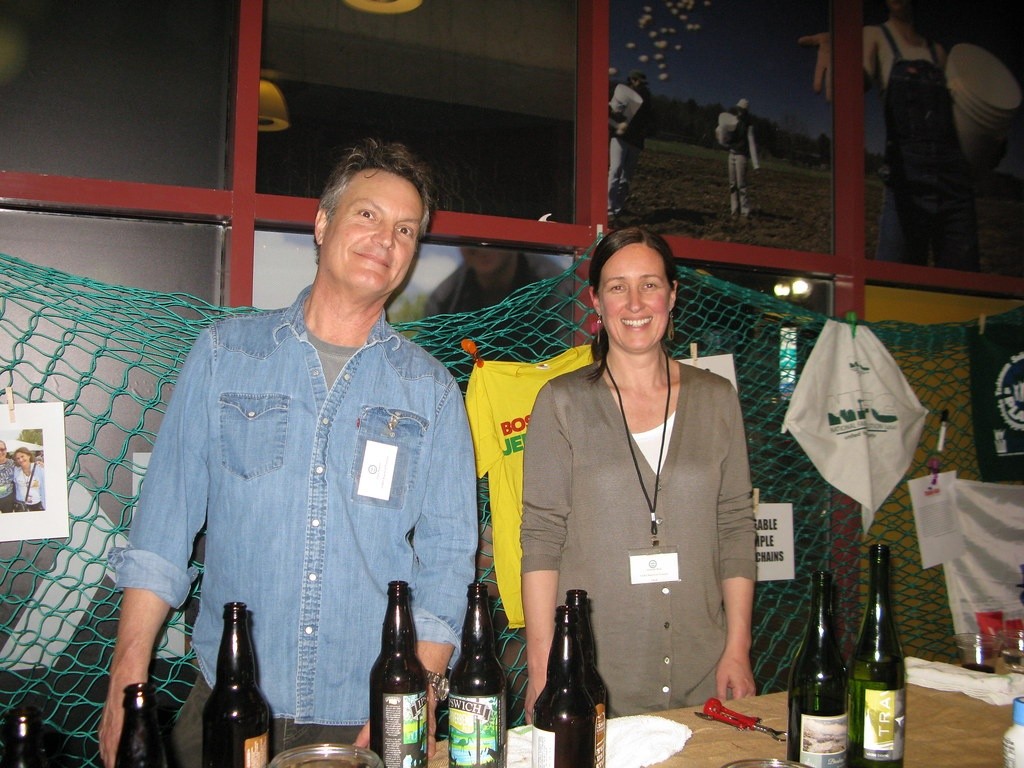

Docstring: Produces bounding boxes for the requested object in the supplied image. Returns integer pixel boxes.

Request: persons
[816,129,831,170]
[417,246,574,403]
[715,98,761,223]
[519,228,756,725]
[0,441,44,513]
[799,0,982,270]
[606,72,650,229]
[100,139,478,768]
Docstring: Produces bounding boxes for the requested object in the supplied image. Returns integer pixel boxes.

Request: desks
[426,657,1024,768]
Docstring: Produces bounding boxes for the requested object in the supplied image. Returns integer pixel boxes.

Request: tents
[0,439,44,453]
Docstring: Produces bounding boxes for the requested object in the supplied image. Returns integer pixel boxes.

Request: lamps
[256,74,292,132]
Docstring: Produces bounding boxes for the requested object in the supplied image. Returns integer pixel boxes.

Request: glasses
[0,448,6,451]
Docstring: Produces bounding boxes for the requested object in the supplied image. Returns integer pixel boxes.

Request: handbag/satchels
[13,500,29,512]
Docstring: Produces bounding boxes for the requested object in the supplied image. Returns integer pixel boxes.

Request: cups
[955,633,1002,673]
[1000,630,1024,676]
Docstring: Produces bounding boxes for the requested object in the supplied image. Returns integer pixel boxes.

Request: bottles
[846,545,907,768]
[6,708,41,768]
[532,604,595,768]
[113,683,164,768]
[788,568,847,768]
[370,581,428,768]
[566,590,607,768]
[1002,696,1024,768]
[448,583,506,768]
[202,601,269,768]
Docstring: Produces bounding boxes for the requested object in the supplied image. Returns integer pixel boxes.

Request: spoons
[694,711,741,731]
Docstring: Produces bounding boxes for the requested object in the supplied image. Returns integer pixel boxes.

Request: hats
[737,98,750,109]
[627,69,648,85]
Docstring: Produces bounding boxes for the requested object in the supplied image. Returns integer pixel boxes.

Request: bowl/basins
[721,760,815,768]
[269,744,383,768]
